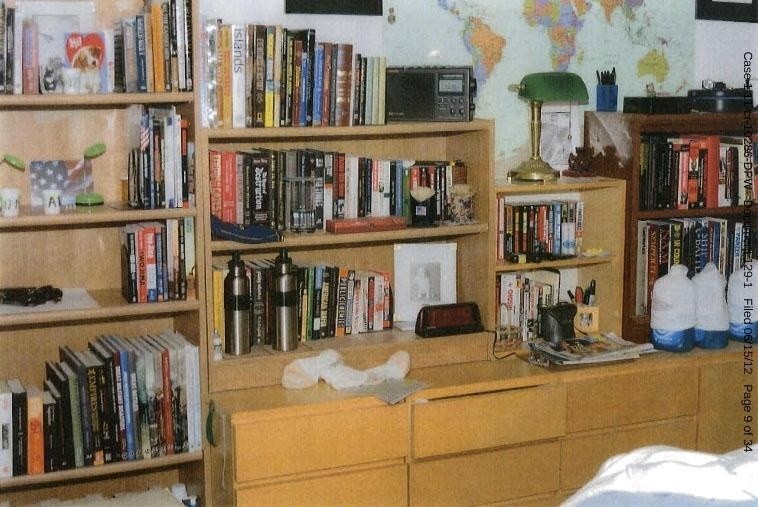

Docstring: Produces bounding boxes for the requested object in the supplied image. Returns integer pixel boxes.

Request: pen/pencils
[597,67,616,85]
[567,279,596,307]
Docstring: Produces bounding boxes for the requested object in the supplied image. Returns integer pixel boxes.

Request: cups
[0,187,23,217]
[41,189,63,215]
[595,83,619,112]
[448,183,475,225]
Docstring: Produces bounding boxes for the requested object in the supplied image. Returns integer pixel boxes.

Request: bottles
[211,329,222,362]
[224,250,250,357]
[272,248,299,353]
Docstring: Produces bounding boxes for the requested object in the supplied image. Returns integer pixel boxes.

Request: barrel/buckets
[727,257,758,344]
[692,262,731,351]
[648,263,697,353]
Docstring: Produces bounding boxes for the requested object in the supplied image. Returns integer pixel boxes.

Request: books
[1,0,758,478]
